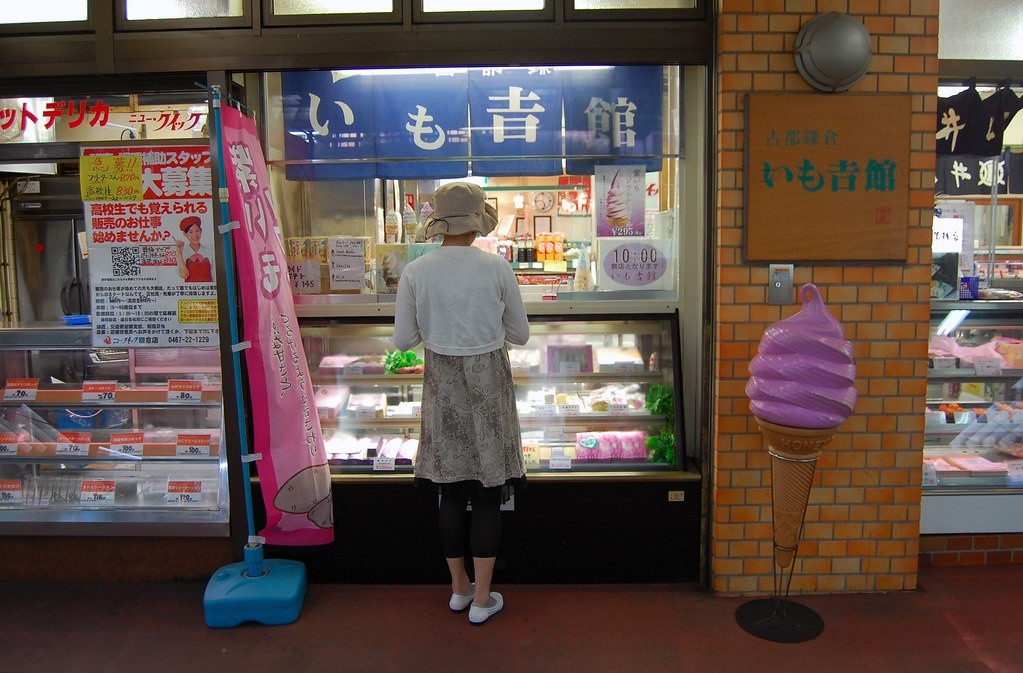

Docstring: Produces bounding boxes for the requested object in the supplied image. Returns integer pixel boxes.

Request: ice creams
[386,201,434,244]
[745,284,858,569]
[606,169,630,228]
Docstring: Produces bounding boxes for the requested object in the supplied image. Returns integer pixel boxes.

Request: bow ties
[189,254,204,263]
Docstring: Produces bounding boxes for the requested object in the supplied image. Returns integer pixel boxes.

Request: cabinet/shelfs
[921,310,1023,495]
[0,346,233,537]
[283,302,689,476]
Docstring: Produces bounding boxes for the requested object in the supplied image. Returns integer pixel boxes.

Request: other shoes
[449,583,475,612]
[469,592,504,625]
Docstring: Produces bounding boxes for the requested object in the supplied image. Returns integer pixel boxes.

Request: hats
[423,181,498,240]
[179,216,201,231]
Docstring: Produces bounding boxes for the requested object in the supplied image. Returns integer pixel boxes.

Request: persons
[394,181,529,622]
[173,216,214,282]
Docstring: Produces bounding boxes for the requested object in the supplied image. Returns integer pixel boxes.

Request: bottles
[518,232,562,262]
